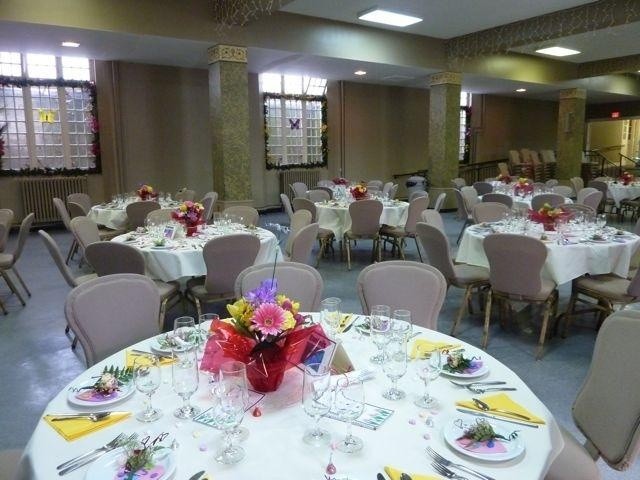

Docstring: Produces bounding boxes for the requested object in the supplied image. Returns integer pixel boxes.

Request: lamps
[534,43,583,57]
[356,6,424,28]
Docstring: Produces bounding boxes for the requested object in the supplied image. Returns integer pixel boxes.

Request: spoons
[473,399,534,421]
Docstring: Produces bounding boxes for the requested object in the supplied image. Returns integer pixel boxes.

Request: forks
[449,379,517,394]
[59,428,138,474]
[45,410,110,422]
[427,449,496,480]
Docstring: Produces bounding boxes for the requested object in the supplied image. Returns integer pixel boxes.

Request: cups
[322,298,342,333]
[320,300,340,342]
[199,313,221,343]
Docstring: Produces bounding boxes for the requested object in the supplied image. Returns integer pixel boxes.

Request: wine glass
[137,363,162,421]
[211,383,246,464]
[173,317,198,368]
[333,377,365,453]
[414,344,441,408]
[489,204,619,242]
[315,182,396,209]
[171,344,201,419]
[302,363,333,446]
[219,360,250,442]
[369,304,392,363]
[106,191,256,248]
[381,335,409,401]
[390,309,413,362]
[487,170,638,200]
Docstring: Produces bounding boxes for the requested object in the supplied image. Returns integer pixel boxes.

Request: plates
[66,375,136,407]
[432,349,489,376]
[150,340,201,353]
[445,415,528,460]
[356,317,412,335]
